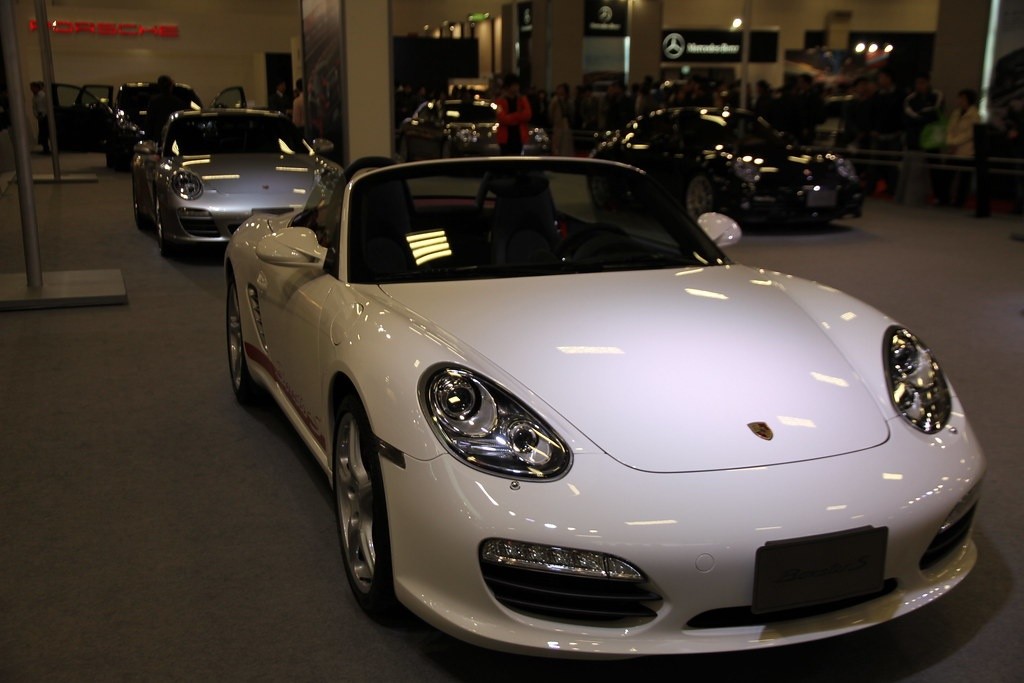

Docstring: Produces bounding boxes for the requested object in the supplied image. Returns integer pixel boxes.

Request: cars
[587,107,866,227]
[51,82,247,172]
[400,99,552,162]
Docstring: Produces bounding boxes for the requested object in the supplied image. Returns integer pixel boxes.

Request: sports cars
[132,108,345,251]
[224,156,986,661]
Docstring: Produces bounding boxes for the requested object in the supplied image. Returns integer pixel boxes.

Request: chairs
[474,161,558,272]
[327,156,425,282]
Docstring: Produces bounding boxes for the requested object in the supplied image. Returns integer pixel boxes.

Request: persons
[573,84,599,131]
[844,76,904,180]
[496,73,532,156]
[292,79,304,127]
[272,80,293,115]
[904,76,1024,217]
[787,74,835,145]
[147,76,179,139]
[528,85,550,127]
[548,83,575,155]
[600,74,772,135]
